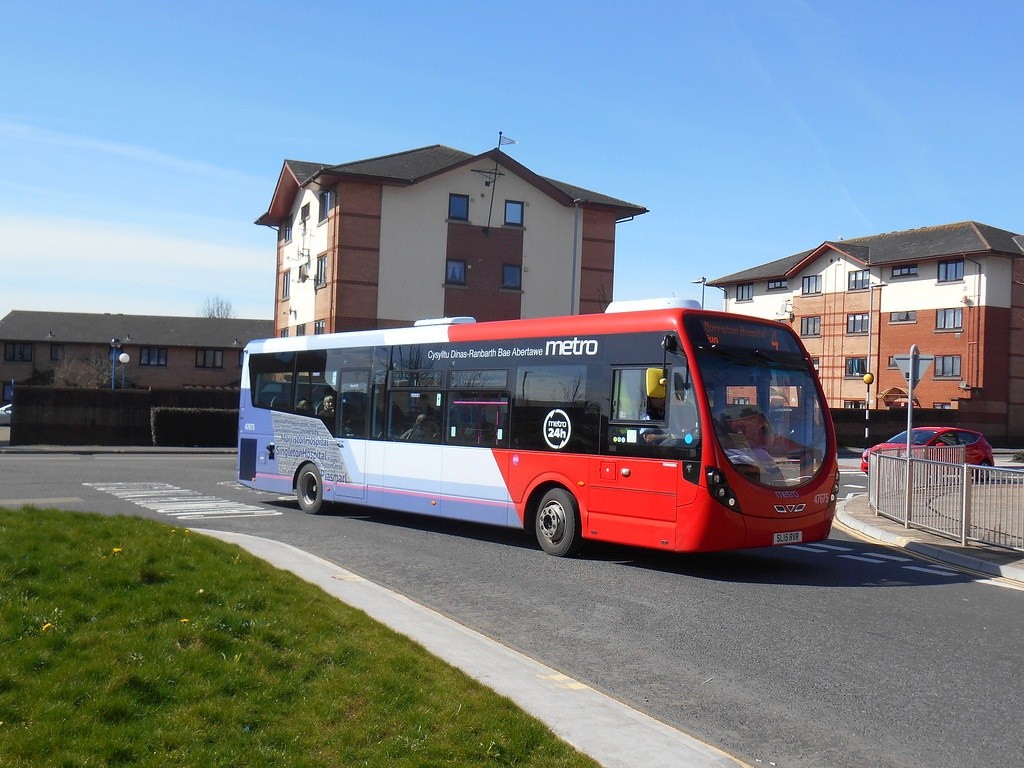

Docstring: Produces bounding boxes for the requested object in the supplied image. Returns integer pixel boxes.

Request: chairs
[315,401,324,415]
[270,394,289,410]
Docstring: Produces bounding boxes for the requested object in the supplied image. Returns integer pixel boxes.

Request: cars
[0,404,12,425]
[860,427,995,483]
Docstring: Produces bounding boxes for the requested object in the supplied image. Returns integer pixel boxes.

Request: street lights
[690,277,707,309]
[119,353,130,389]
[111,339,121,389]
[867,284,888,372]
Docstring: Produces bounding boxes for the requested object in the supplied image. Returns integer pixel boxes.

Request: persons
[409,393,434,441]
[298,395,336,434]
[608,379,679,445]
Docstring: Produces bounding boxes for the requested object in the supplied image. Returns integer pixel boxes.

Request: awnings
[876,387,916,402]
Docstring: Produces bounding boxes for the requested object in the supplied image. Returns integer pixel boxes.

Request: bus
[235,299,840,558]
